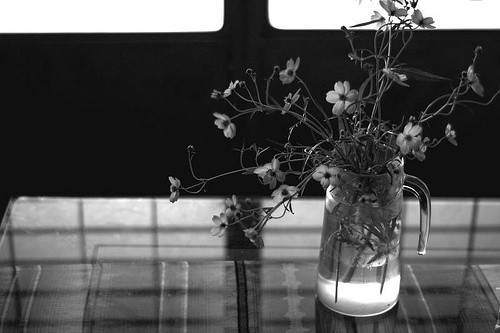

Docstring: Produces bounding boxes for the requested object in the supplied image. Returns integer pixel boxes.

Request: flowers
[167,0,499,249]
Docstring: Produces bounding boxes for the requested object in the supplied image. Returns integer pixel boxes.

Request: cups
[316,145,432,318]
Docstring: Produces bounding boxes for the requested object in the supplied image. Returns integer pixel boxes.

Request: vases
[314,167,433,318]
